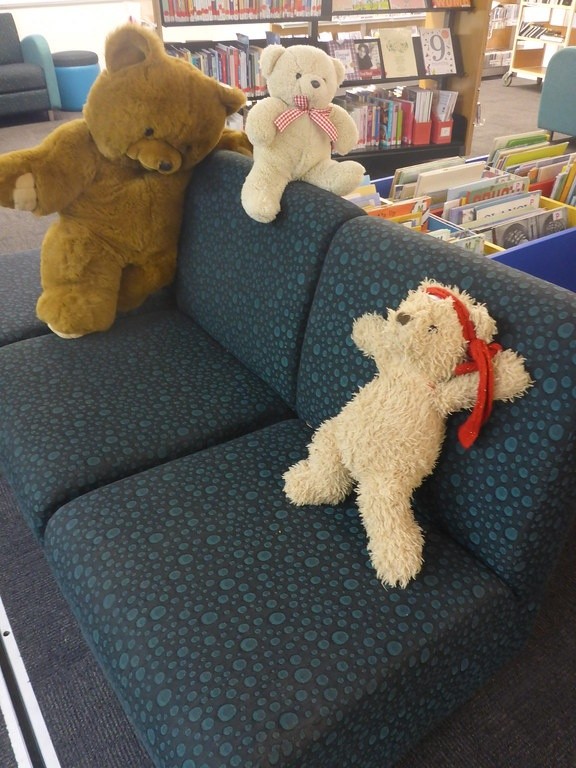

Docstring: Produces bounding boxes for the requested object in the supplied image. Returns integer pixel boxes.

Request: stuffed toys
[240,43,365,224]
[282,278,535,586]
[0,23,253,343]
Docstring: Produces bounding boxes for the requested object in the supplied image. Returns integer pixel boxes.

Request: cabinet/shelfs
[139,0,492,188]
[509,0,576,94]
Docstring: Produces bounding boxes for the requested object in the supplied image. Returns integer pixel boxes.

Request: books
[342,127,575,262]
[153,1,461,155]
[479,0,576,69]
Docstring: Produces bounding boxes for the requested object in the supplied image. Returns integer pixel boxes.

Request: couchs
[0,12,62,126]
[1,151,575,768]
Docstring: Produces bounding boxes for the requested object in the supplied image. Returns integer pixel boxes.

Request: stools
[52,50,102,110]
